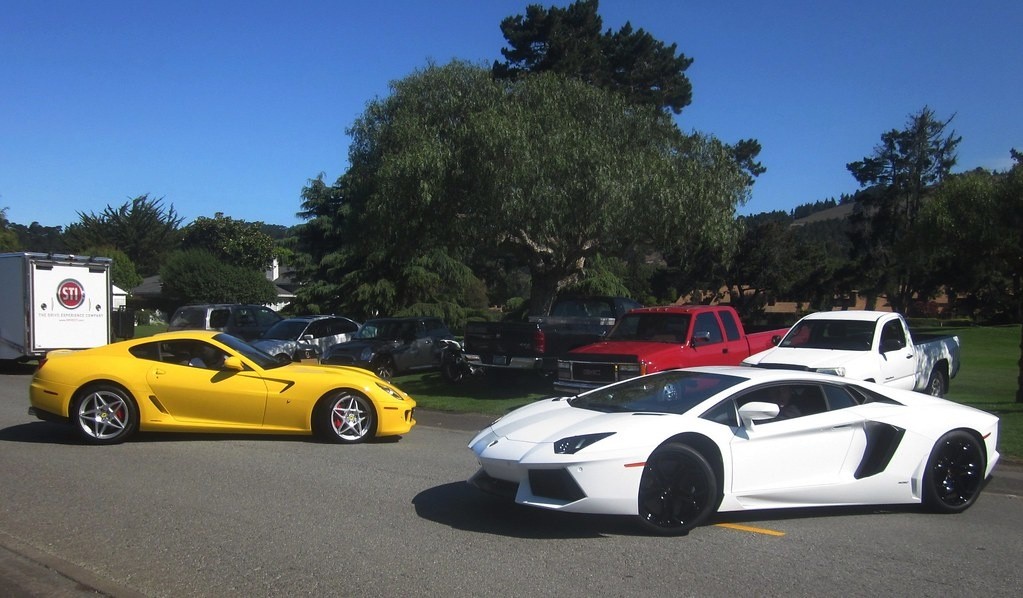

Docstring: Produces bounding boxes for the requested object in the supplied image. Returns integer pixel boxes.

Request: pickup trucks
[552,306,808,404]
[462,293,647,394]
[738,310,962,399]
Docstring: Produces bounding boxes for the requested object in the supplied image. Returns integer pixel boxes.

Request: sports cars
[28,330,418,446]
[464,363,1002,539]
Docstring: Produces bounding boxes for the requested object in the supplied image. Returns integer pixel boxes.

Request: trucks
[0,251,112,364]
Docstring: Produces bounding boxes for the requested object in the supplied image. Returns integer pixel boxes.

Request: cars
[247,314,378,362]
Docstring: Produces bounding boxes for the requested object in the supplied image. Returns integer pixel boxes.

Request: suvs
[322,316,454,381]
[166,303,283,343]
